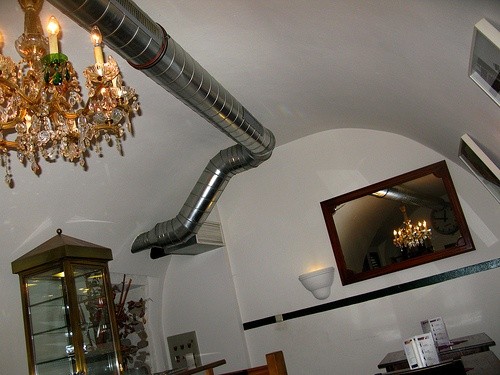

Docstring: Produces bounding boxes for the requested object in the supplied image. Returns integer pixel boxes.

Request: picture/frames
[467,17,500,108]
[458,133,500,204]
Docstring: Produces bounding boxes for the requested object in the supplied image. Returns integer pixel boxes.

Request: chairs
[224,349,289,375]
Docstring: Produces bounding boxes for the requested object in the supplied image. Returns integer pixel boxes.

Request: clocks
[429,203,459,234]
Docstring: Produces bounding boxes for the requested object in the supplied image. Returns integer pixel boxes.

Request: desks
[377,331,496,372]
[375,350,500,375]
[174,359,226,375]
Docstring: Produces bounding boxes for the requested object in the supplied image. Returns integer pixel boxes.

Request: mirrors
[318,159,476,286]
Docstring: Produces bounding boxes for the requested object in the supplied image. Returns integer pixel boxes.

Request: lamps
[297,266,335,300]
[0,0,143,187]
[392,205,434,253]
